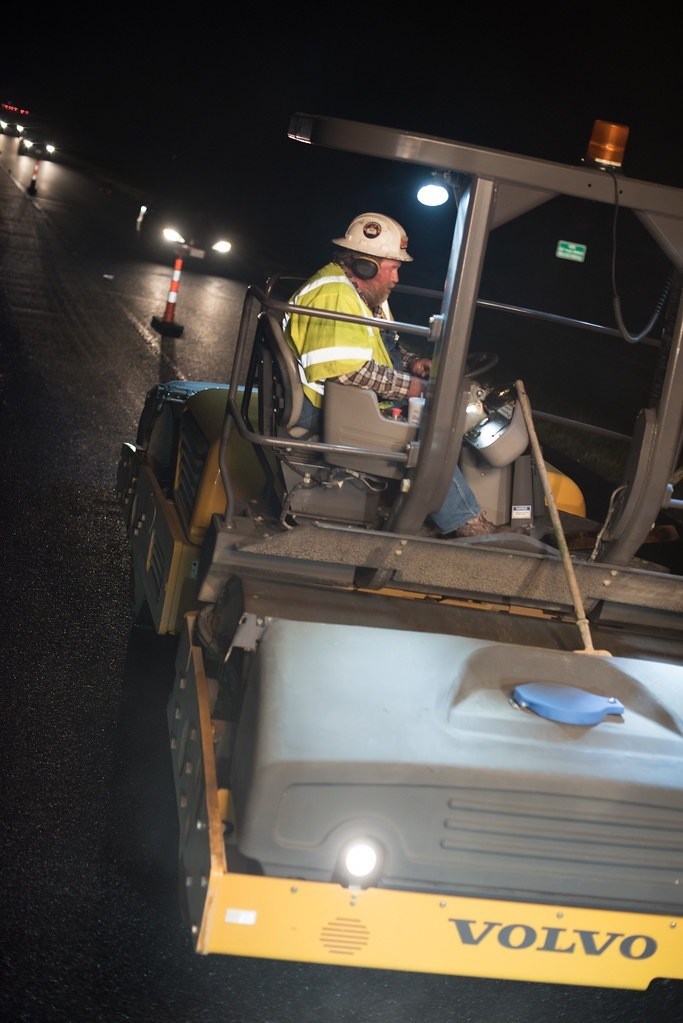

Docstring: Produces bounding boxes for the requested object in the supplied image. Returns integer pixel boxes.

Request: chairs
[260,312,414,483]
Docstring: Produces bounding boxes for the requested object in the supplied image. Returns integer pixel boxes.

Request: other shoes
[447,510,530,538]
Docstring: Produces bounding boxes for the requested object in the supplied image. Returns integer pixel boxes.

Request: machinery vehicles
[113,103,682,1022]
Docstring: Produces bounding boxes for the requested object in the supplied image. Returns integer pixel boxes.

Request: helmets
[332,212,414,261]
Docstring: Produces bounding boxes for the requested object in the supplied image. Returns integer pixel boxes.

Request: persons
[277,213,531,539]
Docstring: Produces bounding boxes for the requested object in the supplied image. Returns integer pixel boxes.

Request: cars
[0,113,59,163]
[137,193,246,271]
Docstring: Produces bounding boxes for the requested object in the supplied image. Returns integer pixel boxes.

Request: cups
[408,397,426,424]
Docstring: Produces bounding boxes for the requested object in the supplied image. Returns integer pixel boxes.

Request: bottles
[387,408,407,423]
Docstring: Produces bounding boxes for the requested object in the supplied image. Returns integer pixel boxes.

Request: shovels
[516,378,612,660]
